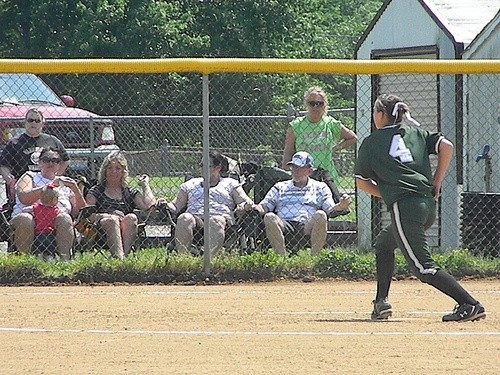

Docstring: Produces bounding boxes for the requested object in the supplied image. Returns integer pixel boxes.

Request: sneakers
[442,300,486,323]
[371,300,392,321]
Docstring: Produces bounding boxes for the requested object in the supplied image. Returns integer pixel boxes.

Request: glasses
[40,156,61,164]
[307,100,325,107]
[26,118,42,123]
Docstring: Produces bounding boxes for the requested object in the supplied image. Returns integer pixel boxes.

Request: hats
[286,150,314,168]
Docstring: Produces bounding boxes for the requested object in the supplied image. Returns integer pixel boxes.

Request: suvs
[0,73,115,179]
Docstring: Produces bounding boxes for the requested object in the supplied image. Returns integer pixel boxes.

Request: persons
[351,94,486,323]
[21,188,71,262]
[86,146,155,264]
[156,153,254,259]
[0,106,71,217]
[253,151,353,261]
[9,147,86,263]
[281,83,357,185]
